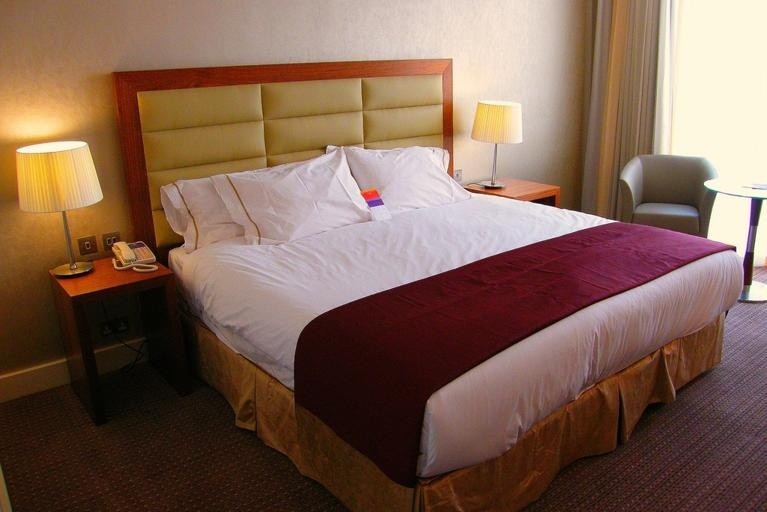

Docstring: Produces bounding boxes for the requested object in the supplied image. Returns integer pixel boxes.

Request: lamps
[469,101,523,189]
[13,140,106,277]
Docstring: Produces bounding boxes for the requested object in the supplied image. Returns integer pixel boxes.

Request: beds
[109,58,743,511]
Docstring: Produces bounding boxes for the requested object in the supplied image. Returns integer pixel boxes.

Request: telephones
[111,240,159,272]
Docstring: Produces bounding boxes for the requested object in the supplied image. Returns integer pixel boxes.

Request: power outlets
[77,230,122,255]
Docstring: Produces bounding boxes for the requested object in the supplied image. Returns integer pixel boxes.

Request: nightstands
[463,177,560,209]
[47,254,196,426]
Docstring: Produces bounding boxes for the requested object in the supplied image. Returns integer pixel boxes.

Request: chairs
[618,154,720,239]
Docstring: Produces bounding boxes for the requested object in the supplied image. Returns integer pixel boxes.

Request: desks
[703,174,766,303]
